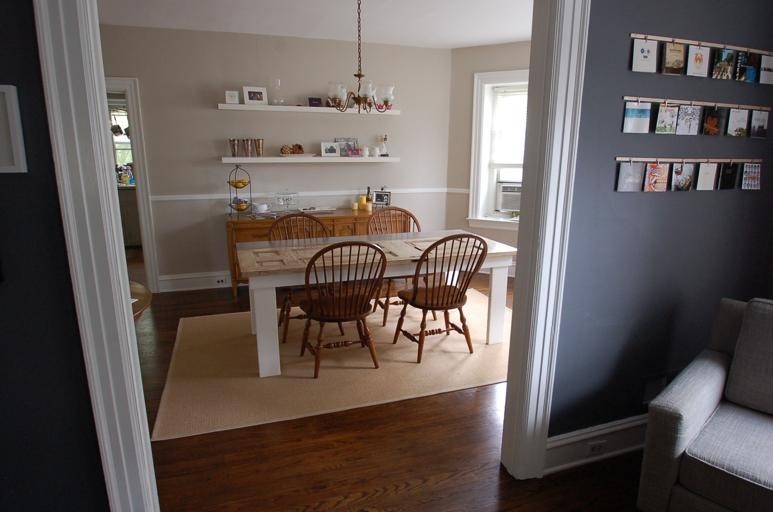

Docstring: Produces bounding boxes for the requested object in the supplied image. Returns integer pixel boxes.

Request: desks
[224,208,411,296]
[129,280,152,322]
[235,229,517,378]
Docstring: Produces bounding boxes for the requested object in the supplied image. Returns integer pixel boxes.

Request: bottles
[375,133,391,157]
[268,76,286,105]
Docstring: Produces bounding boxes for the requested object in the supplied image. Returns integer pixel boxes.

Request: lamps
[327,0,395,114]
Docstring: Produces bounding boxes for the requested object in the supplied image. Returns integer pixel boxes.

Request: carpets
[151,286,513,441]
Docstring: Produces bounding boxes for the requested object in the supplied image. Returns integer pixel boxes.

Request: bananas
[227,180,244,187]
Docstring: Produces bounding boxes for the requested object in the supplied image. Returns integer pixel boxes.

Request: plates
[298,207,336,214]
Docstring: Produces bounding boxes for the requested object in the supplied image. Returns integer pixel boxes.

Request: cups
[253,203,271,213]
[229,137,264,157]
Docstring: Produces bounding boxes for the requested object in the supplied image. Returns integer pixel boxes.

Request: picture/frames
[372,191,391,205]
[242,86,268,104]
[321,142,340,157]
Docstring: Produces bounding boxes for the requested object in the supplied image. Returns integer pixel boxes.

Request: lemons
[235,182,244,188]
[236,204,247,210]
[241,178,248,185]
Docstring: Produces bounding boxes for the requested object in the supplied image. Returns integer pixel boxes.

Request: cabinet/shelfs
[217,103,401,163]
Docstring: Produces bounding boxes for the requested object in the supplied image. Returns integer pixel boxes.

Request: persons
[344,143,353,155]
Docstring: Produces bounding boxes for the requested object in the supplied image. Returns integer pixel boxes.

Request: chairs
[637,297,773,512]
[367,206,437,327]
[269,213,345,343]
[299,241,387,379]
[393,233,488,364]
